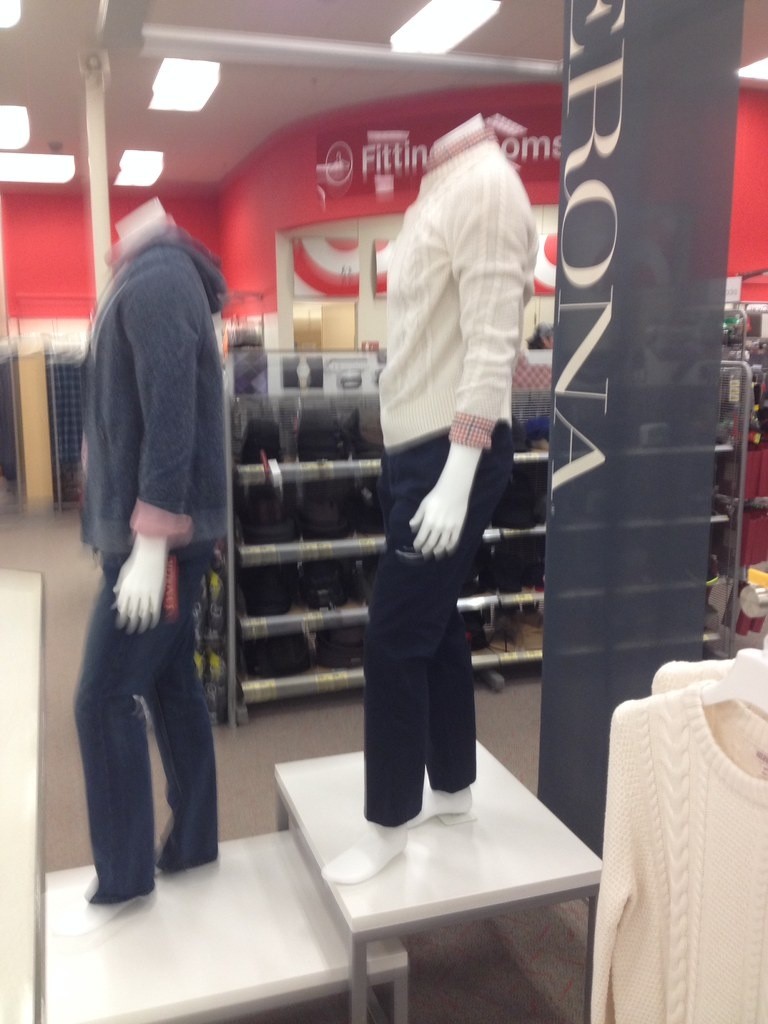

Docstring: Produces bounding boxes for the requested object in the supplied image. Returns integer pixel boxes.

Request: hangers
[612,637,768,730]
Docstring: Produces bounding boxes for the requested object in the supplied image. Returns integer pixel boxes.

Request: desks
[40,738,605,1024]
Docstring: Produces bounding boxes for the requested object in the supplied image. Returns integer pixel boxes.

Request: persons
[528,322,554,350]
[69,198,229,942]
[320,113,536,887]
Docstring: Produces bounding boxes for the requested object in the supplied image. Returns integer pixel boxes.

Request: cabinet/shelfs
[194,344,749,726]
